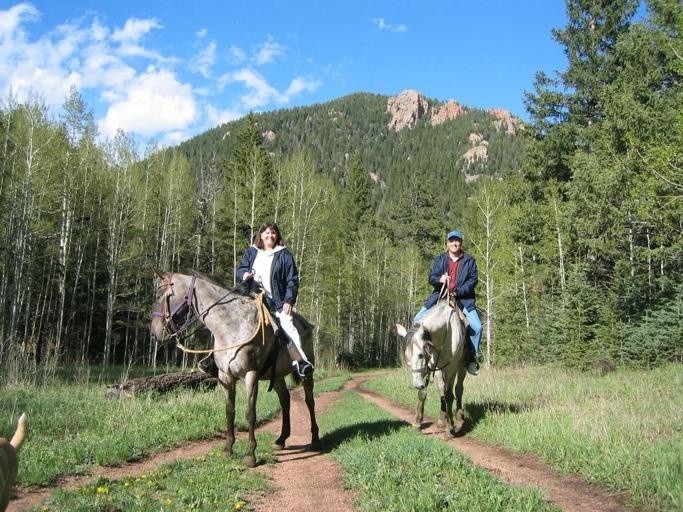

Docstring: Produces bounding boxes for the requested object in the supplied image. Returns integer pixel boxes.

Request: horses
[395,297,474,438]
[149,264,320,469]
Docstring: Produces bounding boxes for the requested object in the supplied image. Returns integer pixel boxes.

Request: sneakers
[299,361,312,374]
[468,361,478,375]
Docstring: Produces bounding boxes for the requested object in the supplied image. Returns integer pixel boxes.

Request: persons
[198,220,315,383]
[411,230,483,375]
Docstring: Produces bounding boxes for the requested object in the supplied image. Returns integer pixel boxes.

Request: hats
[448,231,462,240]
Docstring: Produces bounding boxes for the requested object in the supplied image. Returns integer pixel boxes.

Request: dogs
[0,412,28,512]
